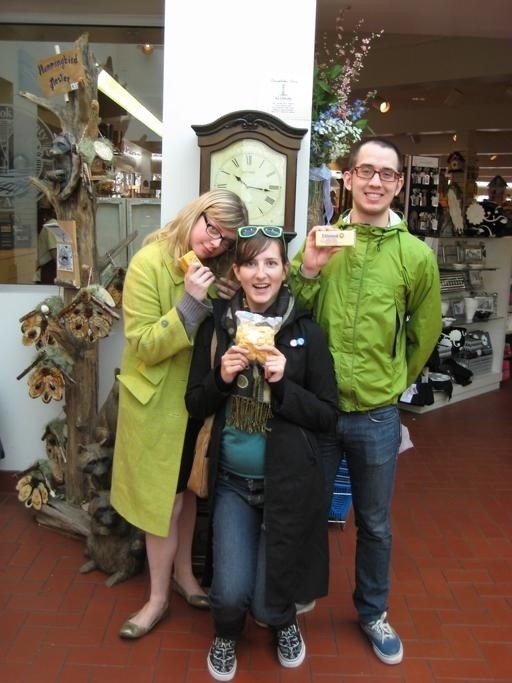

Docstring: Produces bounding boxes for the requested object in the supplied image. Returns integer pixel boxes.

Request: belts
[224,474,260,493]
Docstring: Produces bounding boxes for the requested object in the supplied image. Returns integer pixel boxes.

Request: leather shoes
[171,578,210,608]
[119,609,166,640]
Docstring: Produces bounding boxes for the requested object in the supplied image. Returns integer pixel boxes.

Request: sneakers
[360,617,404,664]
[207,637,237,681]
[257,600,317,669]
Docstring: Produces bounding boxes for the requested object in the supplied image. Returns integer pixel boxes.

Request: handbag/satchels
[187,415,212,499]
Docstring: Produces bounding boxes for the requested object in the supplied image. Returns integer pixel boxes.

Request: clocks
[191,107,309,245]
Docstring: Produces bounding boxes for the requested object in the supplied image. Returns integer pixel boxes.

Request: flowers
[312,5,387,169]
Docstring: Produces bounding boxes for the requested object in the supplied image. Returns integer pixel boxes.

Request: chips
[236,325,273,363]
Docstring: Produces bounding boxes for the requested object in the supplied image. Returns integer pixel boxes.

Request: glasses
[349,166,401,183]
[202,213,235,253]
[236,226,287,259]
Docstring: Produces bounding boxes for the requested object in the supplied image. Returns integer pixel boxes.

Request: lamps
[97,70,162,137]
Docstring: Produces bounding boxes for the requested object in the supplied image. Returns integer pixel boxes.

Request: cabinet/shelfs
[396,236,510,415]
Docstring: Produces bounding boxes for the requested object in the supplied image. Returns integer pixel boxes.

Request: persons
[263,135,446,667]
[172,218,341,681]
[106,183,253,641]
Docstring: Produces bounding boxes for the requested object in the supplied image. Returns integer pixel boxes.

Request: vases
[308,170,326,234]
